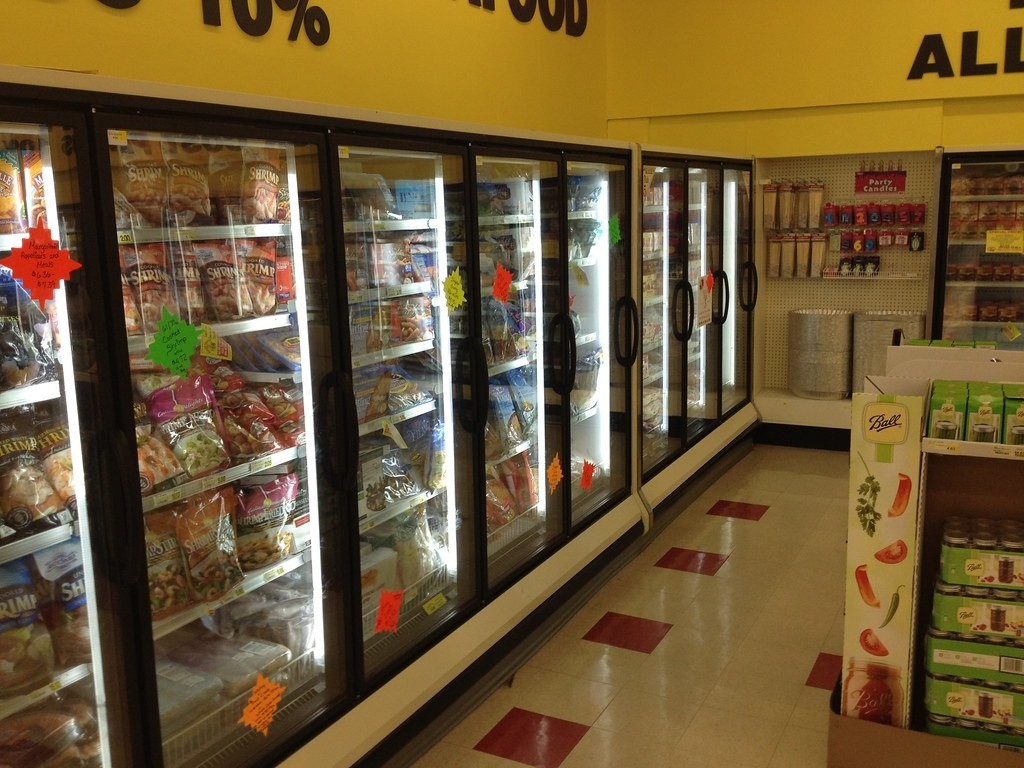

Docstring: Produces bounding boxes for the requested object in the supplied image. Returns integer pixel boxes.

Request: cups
[843,657,903,728]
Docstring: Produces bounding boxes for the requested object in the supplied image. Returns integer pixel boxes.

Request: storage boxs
[826,338,1023,767]
[233,443,398,636]
[944,177,1023,322]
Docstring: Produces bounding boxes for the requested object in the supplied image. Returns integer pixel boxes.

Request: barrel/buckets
[787,307,850,399]
[853,309,925,393]
